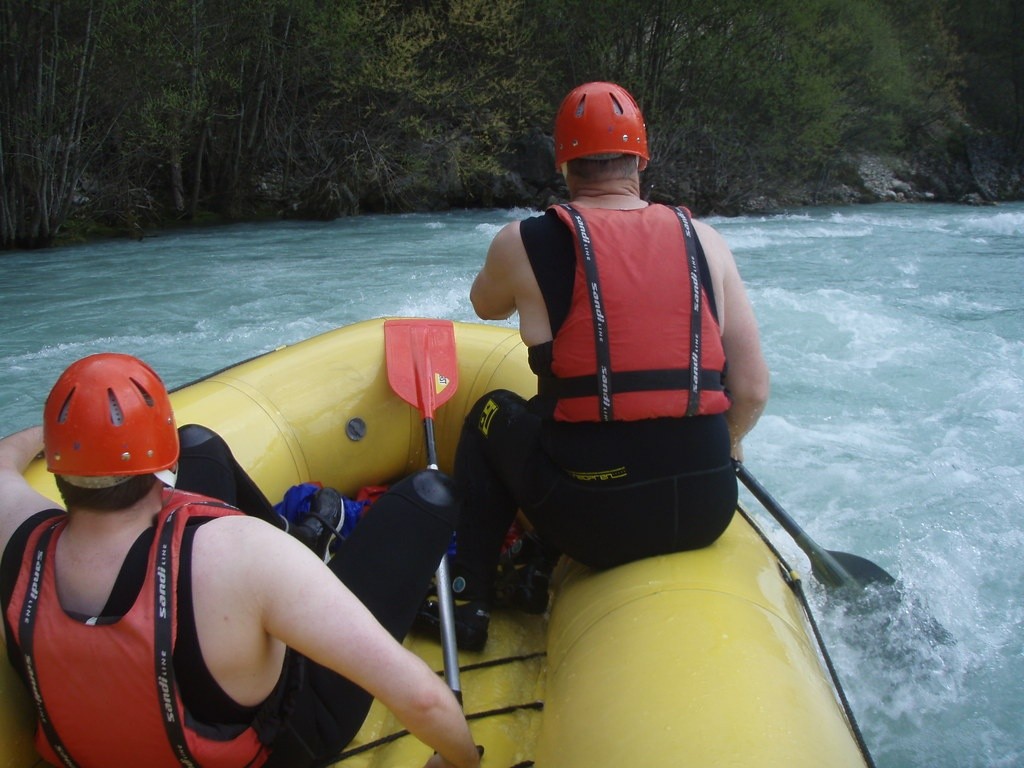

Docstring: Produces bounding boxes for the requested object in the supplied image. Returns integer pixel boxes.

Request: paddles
[728,455,957,680]
[383,316,487,761]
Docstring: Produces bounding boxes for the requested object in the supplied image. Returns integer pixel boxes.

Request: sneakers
[488,533,559,614]
[413,595,490,652]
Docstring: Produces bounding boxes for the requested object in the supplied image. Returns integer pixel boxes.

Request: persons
[414,82,769,653]
[2,354,486,767]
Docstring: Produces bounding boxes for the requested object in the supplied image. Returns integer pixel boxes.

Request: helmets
[555,81,650,172]
[43,353,179,489]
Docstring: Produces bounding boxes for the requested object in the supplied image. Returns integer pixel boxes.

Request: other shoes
[300,486,345,566]
